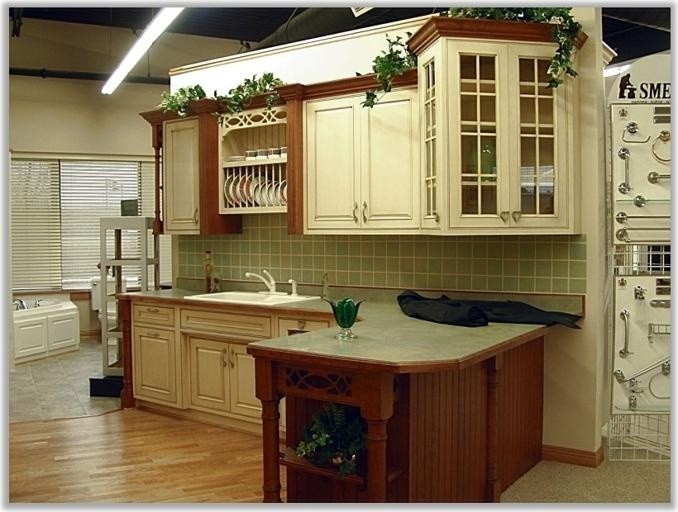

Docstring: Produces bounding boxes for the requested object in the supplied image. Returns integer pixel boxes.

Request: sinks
[183,290,321,307]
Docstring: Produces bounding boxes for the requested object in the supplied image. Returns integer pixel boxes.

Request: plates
[231,172,244,205]
[238,174,246,204]
[254,182,265,206]
[274,183,280,204]
[249,176,265,196]
[266,181,278,205]
[224,172,239,205]
[245,174,253,204]
[279,180,287,202]
[260,180,271,206]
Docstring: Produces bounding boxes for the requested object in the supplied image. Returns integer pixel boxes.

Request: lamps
[100,8,195,95]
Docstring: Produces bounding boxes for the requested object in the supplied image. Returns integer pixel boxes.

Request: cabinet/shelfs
[130,302,338,434]
[302,86,417,230]
[162,114,244,235]
[418,37,570,227]
[218,105,288,215]
[99,218,160,377]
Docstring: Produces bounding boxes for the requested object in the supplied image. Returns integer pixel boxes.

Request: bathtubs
[12,294,64,312]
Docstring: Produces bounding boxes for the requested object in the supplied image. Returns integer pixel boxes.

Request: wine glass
[321,296,368,340]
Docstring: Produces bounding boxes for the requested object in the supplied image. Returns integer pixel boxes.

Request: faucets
[13,297,29,309]
[244,270,276,293]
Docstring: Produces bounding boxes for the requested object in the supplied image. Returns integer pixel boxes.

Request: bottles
[204,250,213,292]
[246,146,287,160]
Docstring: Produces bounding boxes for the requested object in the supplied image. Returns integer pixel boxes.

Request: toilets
[90,275,128,345]
